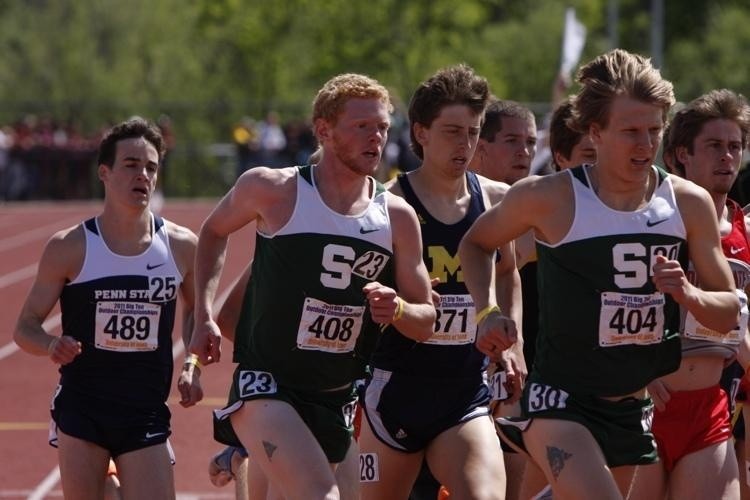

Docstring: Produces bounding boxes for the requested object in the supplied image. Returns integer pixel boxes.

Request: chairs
[209,446,237,488]
[107,457,117,476]
[437,485,450,500]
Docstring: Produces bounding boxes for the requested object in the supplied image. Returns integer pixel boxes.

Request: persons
[629,88,750,499]
[13,116,202,499]
[334,63,599,500]
[456,46,740,498]
[189,71,437,500]
[1,116,175,200]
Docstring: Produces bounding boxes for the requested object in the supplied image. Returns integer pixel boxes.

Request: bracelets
[395,296,403,320]
[183,358,201,372]
[477,304,501,324]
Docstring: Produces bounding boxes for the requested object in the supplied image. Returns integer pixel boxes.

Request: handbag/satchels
[183,358,201,372]
